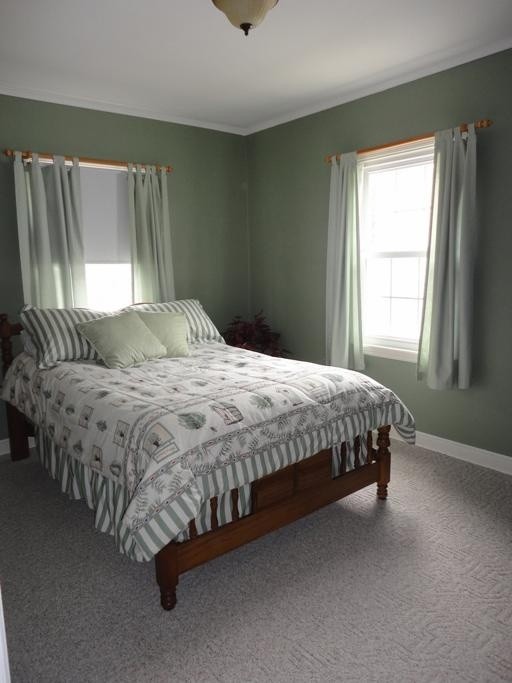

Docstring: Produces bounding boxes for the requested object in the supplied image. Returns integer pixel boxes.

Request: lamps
[211,1,279,37]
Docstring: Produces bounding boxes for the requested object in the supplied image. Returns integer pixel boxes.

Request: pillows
[21,298,224,370]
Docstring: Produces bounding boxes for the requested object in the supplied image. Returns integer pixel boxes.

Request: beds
[1,298,392,610]
